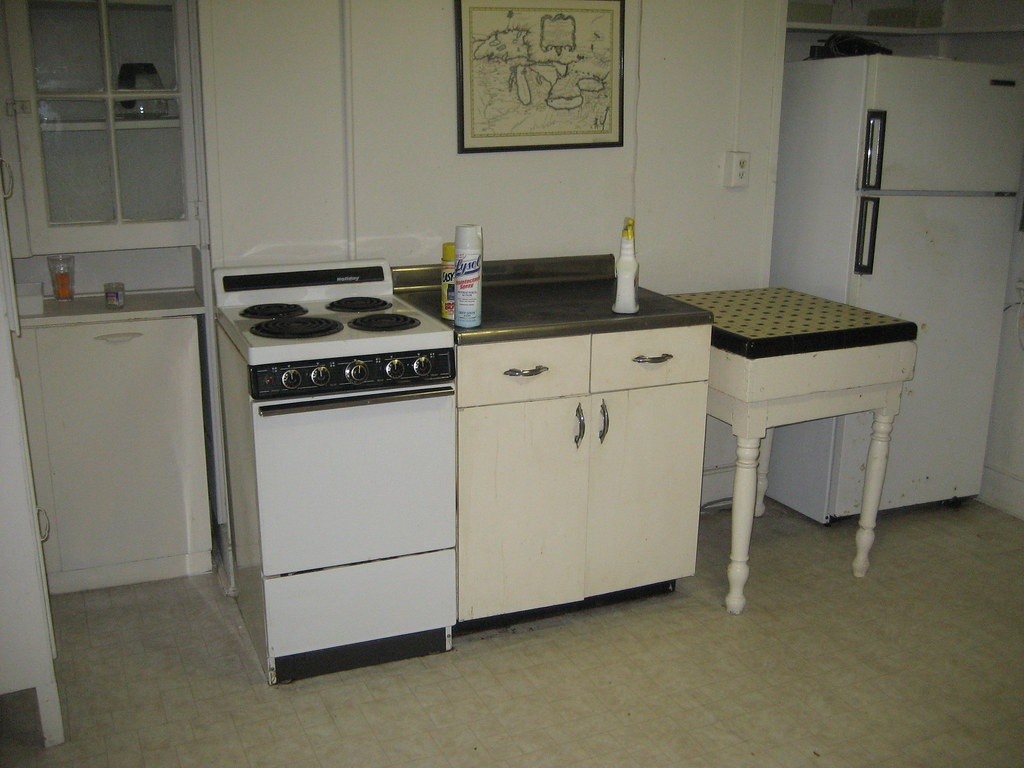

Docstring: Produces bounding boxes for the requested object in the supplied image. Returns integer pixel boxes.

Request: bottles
[453,225,483,328]
[440,243,456,321]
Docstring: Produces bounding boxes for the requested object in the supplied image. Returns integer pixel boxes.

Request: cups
[47,255,75,302]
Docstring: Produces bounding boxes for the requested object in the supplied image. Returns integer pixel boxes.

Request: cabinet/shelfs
[0,0,220,596]
[456,325,712,621]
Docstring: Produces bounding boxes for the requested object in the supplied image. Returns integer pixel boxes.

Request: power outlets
[725,150,749,189]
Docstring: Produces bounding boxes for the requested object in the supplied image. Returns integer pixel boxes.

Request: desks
[670,288,917,614]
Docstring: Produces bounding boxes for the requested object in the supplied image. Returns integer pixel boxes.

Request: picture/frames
[450,0,626,153]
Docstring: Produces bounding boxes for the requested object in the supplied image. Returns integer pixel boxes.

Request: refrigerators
[762,52,1024,529]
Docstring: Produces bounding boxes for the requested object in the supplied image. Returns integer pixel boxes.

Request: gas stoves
[210,257,457,400]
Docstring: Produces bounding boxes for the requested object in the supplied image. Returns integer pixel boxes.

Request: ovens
[219,383,456,685]
